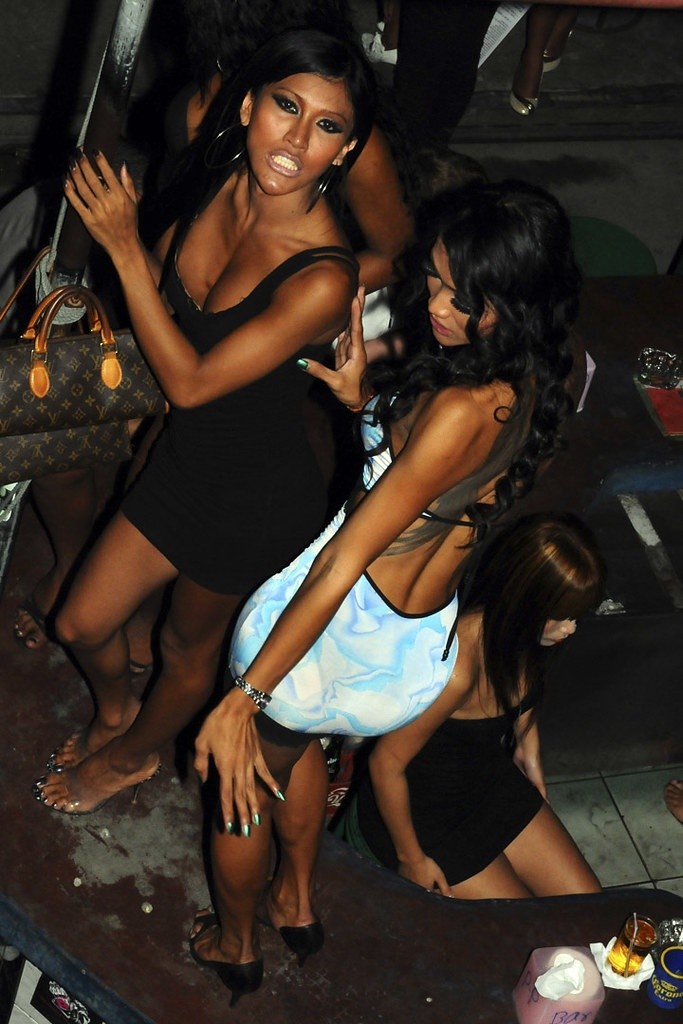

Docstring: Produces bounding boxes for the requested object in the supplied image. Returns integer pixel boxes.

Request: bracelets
[349,407,362,411]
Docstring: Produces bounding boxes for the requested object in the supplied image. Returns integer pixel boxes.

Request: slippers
[14,592,54,650]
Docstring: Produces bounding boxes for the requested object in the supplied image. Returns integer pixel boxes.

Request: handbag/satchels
[0,415,134,483]
[0,285,169,438]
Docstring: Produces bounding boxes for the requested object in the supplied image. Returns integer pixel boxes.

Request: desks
[479,275,683,782]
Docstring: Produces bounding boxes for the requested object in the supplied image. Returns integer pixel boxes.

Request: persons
[510,4,581,115]
[387,140,488,213]
[397,0,500,145]
[189,186,597,1007]
[665,780,683,825]
[0,0,417,816]
[355,510,603,898]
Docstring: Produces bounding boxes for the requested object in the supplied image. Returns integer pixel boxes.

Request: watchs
[235,677,271,710]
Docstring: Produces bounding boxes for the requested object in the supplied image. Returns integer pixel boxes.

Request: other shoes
[509,73,543,115]
[541,52,560,72]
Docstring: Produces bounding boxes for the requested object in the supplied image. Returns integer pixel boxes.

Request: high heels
[259,885,324,969]
[187,909,265,1006]
[46,699,148,771]
[33,744,164,815]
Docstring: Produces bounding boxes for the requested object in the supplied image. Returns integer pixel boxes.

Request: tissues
[512,944,607,1024]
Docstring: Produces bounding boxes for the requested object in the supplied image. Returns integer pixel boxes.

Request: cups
[646,945,683,1010]
[607,912,660,977]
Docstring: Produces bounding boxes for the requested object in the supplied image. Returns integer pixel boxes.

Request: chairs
[570,216,657,275]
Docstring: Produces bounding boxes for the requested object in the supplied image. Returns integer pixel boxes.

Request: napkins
[590,936,656,991]
